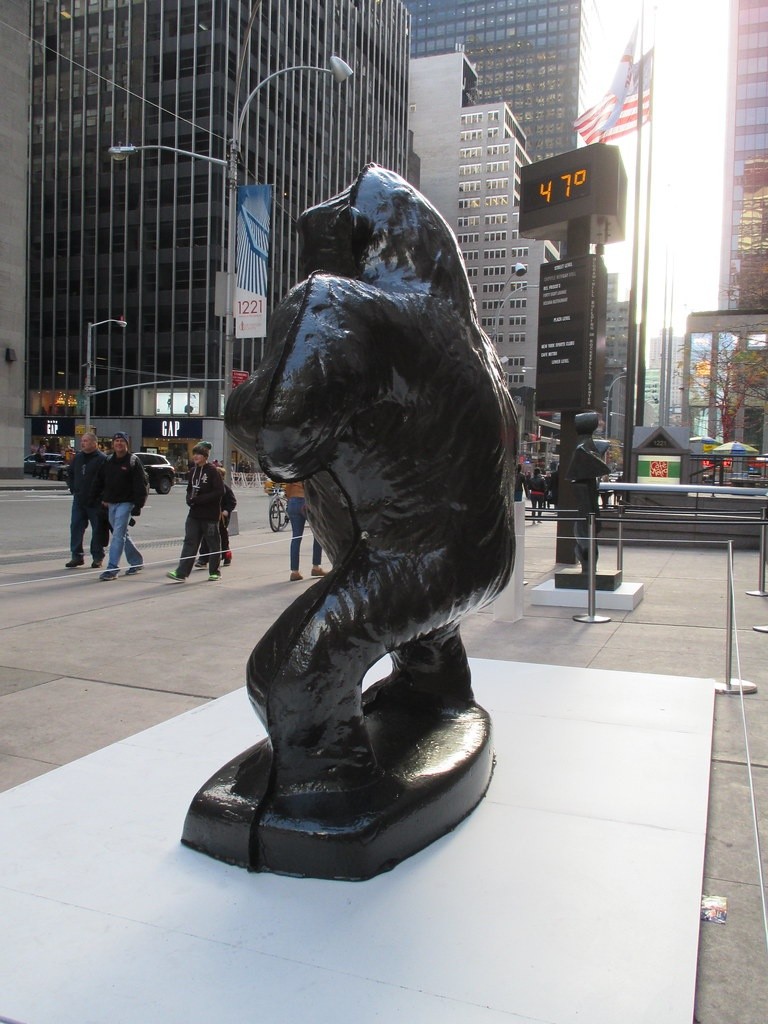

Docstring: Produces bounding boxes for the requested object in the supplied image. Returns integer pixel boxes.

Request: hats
[112,431,129,444]
[193,441,213,459]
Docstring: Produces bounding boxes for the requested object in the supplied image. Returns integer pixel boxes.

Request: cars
[262,475,289,497]
[24,452,66,476]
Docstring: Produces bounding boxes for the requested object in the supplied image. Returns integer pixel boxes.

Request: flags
[572,49,652,147]
[599,18,638,134]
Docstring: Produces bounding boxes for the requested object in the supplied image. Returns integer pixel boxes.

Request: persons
[528,468,558,524]
[238,460,251,473]
[195,467,237,569]
[526,472,531,480]
[166,441,226,581]
[600,476,612,505]
[31,448,42,478]
[287,481,326,581]
[99,431,149,580]
[514,464,529,502]
[616,476,622,502]
[64,432,109,567]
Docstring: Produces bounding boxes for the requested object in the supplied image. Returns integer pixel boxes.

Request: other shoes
[311,568,327,576]
[289,573,303,580]
[65,558,85,567]
[91,560,103,568]
[223,559,232,566]
[167,571,186,582]
[99,571,119,581]
[538,520,542,523]
[532,521,536,525]
[207,574,222,581]
[124,565,144,575]
[194,562,208,569]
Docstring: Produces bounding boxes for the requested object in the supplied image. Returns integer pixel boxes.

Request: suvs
[101,451,177,494]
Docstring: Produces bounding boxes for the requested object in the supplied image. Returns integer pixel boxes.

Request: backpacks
[129,451,151,508]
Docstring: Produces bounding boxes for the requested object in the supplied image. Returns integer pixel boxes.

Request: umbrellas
[689,436,722,446]
[713,441,760,455]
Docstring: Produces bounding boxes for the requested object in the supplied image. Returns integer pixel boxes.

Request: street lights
[106,143,243,505]
[87,319,127,430]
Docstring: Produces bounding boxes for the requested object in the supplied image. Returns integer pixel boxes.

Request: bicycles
[268,481,292,533]
